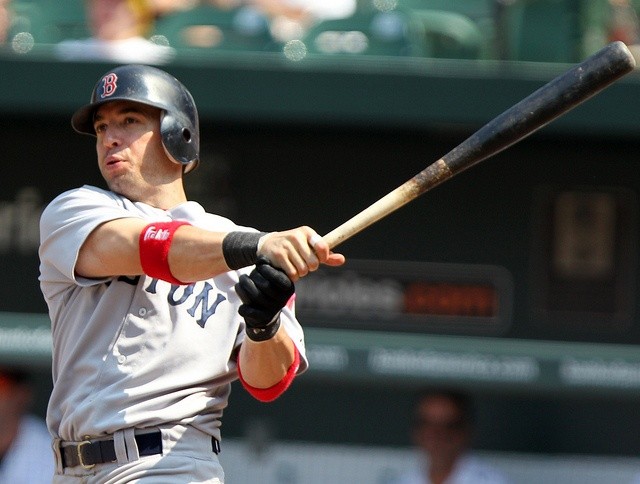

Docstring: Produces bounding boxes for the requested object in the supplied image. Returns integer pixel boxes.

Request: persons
[1,363,56,484]
[388,385,507,484]
[37,64,345,484]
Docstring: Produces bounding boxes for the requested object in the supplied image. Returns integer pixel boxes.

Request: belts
[59,433,220,468]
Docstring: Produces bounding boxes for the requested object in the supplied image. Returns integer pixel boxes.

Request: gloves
[235,263,296,342]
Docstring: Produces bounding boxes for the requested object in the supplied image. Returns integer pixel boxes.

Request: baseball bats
[235,41,635,304]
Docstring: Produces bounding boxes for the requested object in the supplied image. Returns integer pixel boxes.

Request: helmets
[71,64,199,172]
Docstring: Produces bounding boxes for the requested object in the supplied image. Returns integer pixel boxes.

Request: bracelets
[245,313,281,342]
[222,229,269,271]
[236,340,300,402]
[139,220,196,286]
